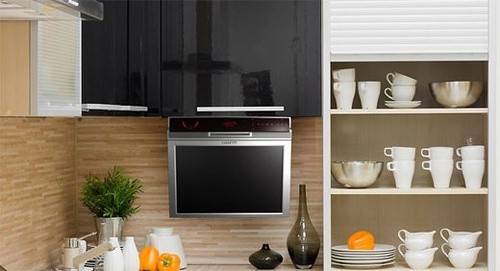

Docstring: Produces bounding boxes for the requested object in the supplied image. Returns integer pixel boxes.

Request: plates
[385,101,422,108]
[332,244,396,268]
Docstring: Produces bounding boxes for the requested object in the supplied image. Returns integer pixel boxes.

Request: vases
[248,243,284,270]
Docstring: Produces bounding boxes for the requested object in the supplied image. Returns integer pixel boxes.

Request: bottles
[103,237,123,271]
[122,237,140,271]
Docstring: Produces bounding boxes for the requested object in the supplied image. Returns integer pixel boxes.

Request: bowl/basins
[429,80,485,107]
[332,161,384,188]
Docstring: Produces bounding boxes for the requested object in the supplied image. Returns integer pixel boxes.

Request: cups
[383,147,416,161]
[358,81,381,109]
[332,68,356,81]
[332,82,356,109]
[421,159,454,189]
[456,146,485,160]
[456,161,485,188]
[384,86,416,101]
[421,147,454,159]
[386,161,415,188]
[386,72,417,86]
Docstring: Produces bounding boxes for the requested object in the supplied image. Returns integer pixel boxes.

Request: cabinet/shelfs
[1,10,83,119]
[321,3,495,271]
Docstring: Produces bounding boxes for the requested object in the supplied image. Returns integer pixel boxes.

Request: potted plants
[81,163,147,246]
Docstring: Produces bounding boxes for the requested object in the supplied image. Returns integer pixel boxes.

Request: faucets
[54,231,117,271]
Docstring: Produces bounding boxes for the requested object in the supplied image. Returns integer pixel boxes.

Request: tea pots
[441,242,483,269]
[397,229,436,250]
[440,228,483,250]
[397,243,439,268]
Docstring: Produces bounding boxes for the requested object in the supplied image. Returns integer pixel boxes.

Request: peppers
[139,245,159,271]
[157,252,181,271]
[347,230,376,251]
